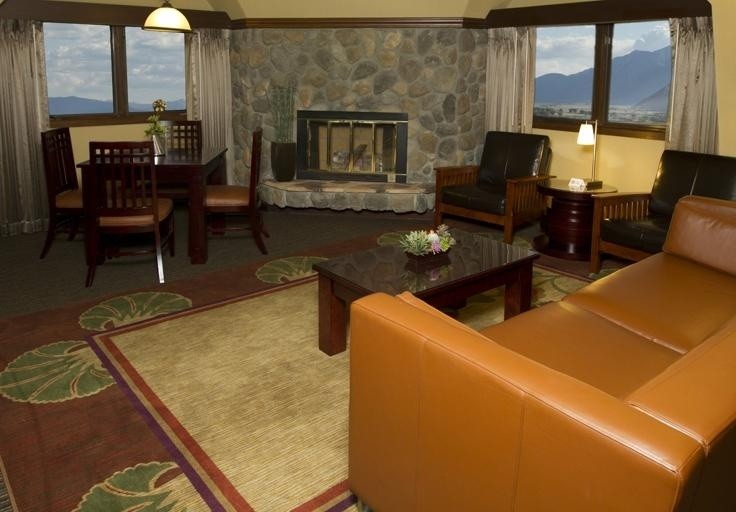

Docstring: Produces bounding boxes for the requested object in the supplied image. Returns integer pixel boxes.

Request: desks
[73,147,230,268]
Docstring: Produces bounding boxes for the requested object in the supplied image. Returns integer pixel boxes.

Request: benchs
[590,146,735,281]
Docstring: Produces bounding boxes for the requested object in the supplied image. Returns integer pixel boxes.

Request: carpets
[1,229,601,512]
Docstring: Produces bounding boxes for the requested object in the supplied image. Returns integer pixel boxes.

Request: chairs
[433,129,558,246]
[81,137,178,291]
[37,127,85,256]
[205,124,271,253]
[159,121,202,155]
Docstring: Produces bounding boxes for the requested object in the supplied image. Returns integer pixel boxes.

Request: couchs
[342,193,736,512]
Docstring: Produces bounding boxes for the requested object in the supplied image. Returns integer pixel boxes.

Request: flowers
[144,97,167,135]
[399,222,458,256]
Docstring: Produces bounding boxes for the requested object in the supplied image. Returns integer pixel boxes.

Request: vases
[268,140,296,184]
[150,130,166,156]
[406,249,450,260]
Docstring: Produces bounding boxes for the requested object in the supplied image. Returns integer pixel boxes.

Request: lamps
[141,2,195,37]
[576,117,605,188]
